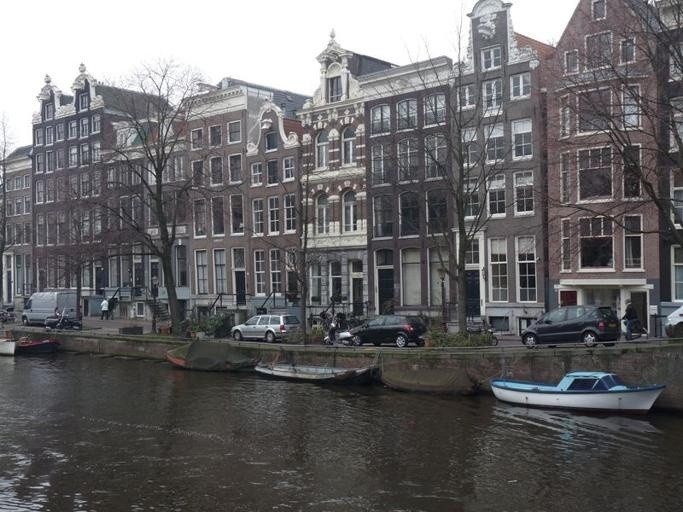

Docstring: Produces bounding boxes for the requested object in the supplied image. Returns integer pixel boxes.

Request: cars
[664,305,682,339]
[0,309,16,322]
[520,305,619,349]
[349,315,426,347]
[230,313,301,342]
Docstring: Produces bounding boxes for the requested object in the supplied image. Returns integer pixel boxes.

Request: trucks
[21,291,82,326]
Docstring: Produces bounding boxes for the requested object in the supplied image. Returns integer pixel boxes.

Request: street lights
[150,275,161,335]
[436,267,447,332]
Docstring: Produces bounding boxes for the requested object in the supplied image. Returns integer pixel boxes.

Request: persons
[108,298,115,320]
[100,298,109,321]
[621,299,638,341]
[346,313,361,332]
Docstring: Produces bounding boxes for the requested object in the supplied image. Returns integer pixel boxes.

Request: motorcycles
[321,317,352,345]
[54,307,81,330]
[468,320,497,346]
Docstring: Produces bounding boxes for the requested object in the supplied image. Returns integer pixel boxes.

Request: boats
[254,363,377,386]
[165,338,255,371]
[491,405,662,436]
[488,370,666,418]
[0,336,61,357]
[381,364,482,397]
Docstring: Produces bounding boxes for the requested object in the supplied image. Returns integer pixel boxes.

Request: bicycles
[615,318,648,343]
